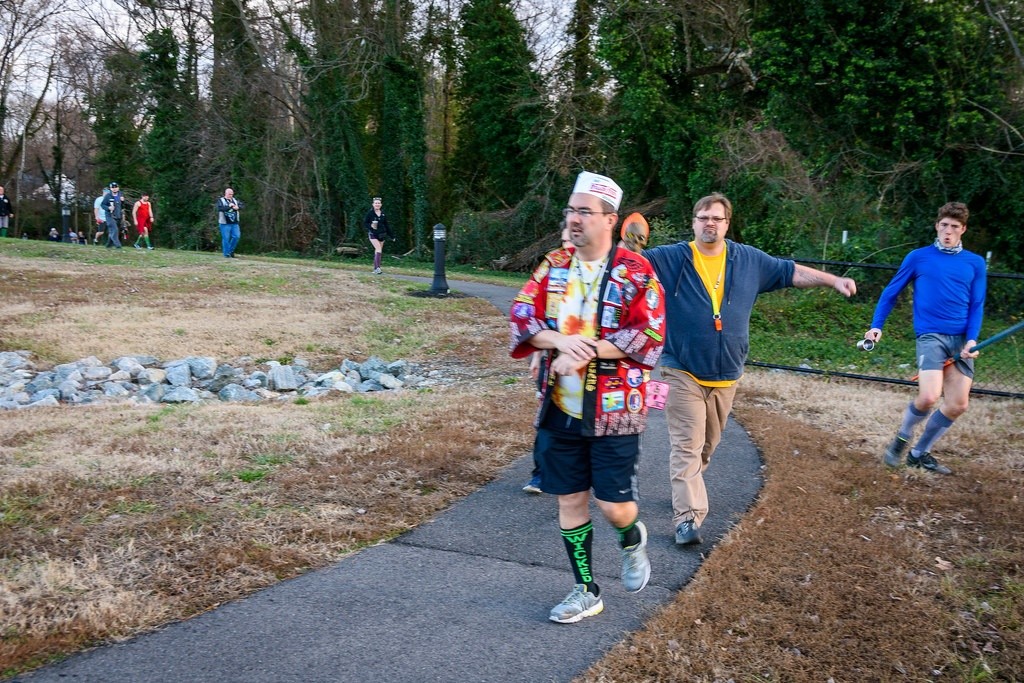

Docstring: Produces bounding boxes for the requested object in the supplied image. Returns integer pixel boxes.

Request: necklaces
[574,251,607,322]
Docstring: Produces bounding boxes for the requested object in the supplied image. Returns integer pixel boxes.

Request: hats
[573,171,623,211]
[109,182,118,187]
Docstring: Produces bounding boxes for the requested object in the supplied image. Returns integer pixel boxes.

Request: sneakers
[621,521,651,594]
[522,473,544,493]
[907,451,951,473]
[675,518,703,544]
[549,582,604,622]
[884,436,907,467]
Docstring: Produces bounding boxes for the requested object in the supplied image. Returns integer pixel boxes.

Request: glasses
[694,215,728,222]
[111,185,117,188]
[562,208,613,217]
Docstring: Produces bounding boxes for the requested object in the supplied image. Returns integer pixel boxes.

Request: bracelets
[589,344,599,357]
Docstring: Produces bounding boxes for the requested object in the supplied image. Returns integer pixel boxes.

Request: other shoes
[134,244,140,248]
[147,246,155,250]
[375,267,382,274]
[225,252,235,258]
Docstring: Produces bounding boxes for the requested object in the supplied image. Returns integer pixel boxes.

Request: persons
[132,191,154,250]
[47,228,60,241]
[101,182,128,249]
[864,201,987,476]
[522,219,574,494]
[215,188,245,257]
[364,198,388,275]
[93,187,110,245]
[511,172,666,624]
[65,226,87,245]
[0,186,14,237]
[638,193,856,545]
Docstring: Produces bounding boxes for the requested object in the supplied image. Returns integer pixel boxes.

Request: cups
[373,221,378,229]
[857,339,875,352]
